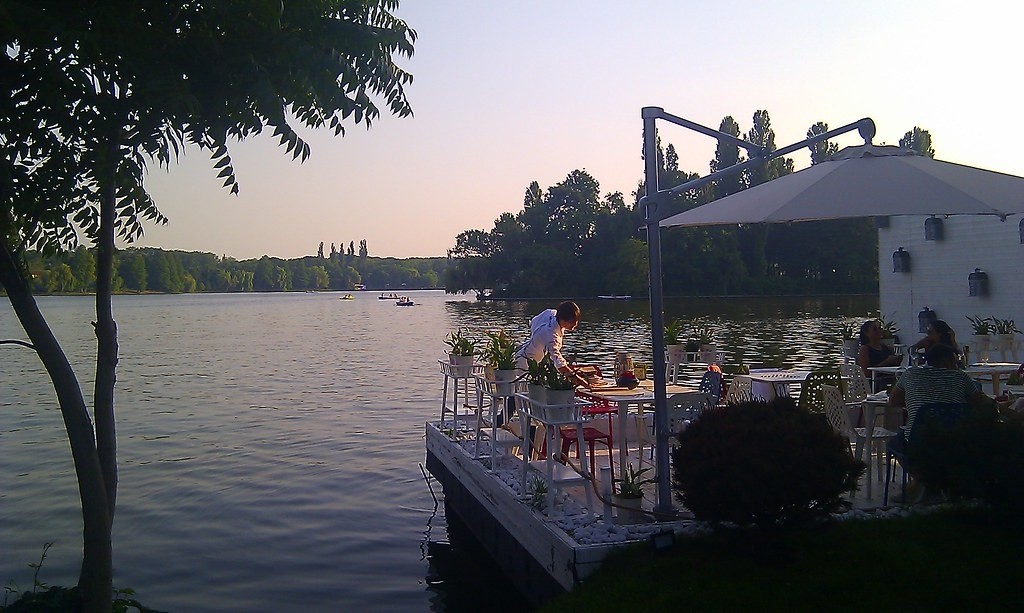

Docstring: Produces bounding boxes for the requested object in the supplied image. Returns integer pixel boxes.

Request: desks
[867,365,1019,395]
[736,371,871,402]
[574,378,718,486]
[862,394,997,502]
[1004,382,1024,401]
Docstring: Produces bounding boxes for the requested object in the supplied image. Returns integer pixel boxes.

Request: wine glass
[910,348,918,368]
[982,352,989,367]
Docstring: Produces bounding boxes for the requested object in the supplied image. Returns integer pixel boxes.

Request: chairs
[543,363,995,511]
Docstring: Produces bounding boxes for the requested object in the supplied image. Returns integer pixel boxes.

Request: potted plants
[612,462,654,516]
[443,325,485,377]
[482,329,527,394]
[664,320,686,362]
[838,319,861,357]
[875,315,900,346]
[697,330,718,362]
[520,345,590,422]
[965,314,1023,349]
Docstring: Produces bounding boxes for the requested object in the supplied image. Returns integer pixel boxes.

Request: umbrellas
[637,104,1024,519]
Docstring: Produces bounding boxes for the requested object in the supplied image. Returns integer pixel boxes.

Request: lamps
[600,466,611,495]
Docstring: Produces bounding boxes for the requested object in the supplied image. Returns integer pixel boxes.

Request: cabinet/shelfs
[472,371,534,473]
[515,394,594,518]
[973,348,1018,383]
[439,358,485,442]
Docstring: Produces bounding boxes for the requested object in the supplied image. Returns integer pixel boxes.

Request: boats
[339,297,355,300]
[379,297,400,299]
[396,302,414,306]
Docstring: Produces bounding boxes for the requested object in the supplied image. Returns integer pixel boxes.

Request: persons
[889,345,1001,505]
[859,320,904,394]
[912,320,968,371]
[494,300,595,464]
[341,294,353,300]
[379,292,411,303]
